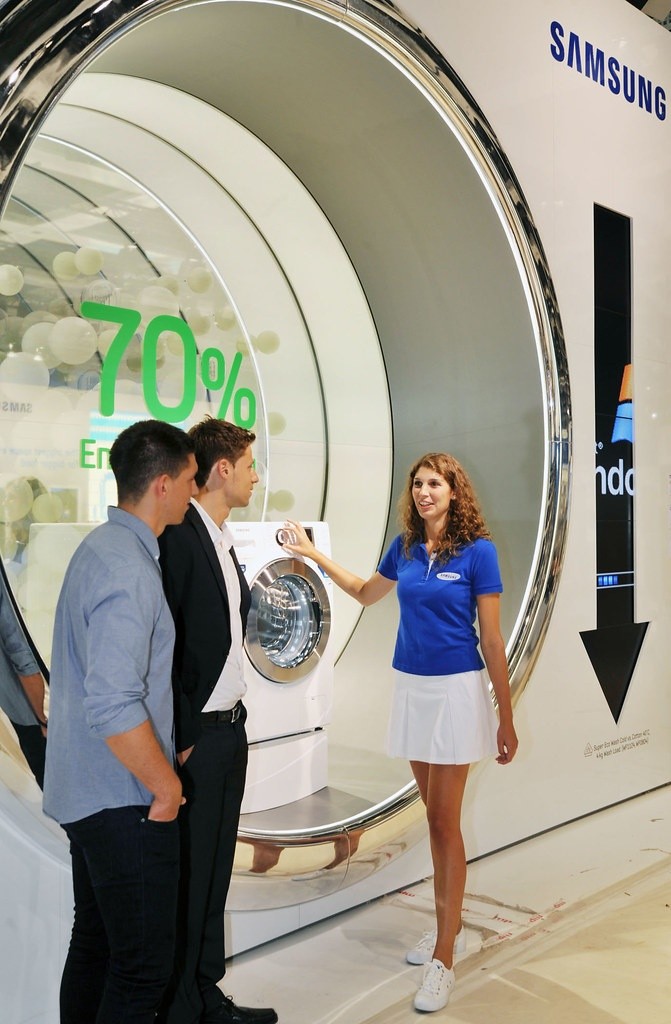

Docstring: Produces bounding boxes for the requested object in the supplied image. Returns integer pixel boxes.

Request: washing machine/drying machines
[216,521,331,814]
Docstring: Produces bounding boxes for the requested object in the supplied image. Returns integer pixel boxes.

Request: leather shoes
[203,995,277,1024]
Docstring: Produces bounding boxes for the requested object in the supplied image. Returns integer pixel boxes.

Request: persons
[0,555,50,790]
[158,418,278,1024]
[43,421,200,1024]
[282,453,518,1012]
[0,473,68,580]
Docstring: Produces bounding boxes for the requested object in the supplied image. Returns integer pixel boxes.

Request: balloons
[0,247,281,403]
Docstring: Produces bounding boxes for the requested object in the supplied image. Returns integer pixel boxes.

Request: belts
[199,705,243,725]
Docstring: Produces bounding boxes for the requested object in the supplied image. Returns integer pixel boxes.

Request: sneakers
[406,925,467,964]
[292,869,330,882]
[413,959,455,1013]
[233,870,269,876]
